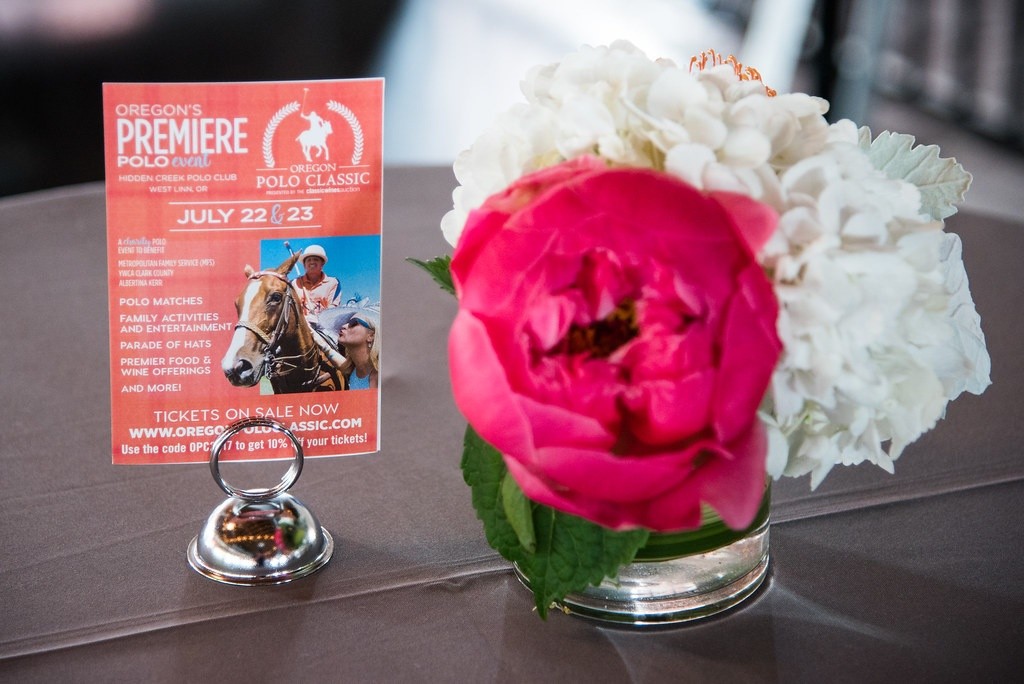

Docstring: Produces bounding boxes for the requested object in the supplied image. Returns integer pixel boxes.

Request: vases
[513,484,777,636]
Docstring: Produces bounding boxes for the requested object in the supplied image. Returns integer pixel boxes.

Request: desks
[0,166,1024,684]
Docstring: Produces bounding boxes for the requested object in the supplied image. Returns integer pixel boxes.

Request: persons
[306,313,378,391]
[290,244,342,323]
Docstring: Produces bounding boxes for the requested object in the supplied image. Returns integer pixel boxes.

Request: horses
[221,247,350,394]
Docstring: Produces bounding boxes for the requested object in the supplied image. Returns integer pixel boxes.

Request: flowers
[403,38,996,617]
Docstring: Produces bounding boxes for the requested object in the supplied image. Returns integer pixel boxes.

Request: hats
[298,245,328,265]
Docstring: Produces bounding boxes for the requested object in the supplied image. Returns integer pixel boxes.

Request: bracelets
[323,344,336,359]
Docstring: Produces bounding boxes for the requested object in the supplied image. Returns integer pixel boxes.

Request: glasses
[346,318,372,330]
